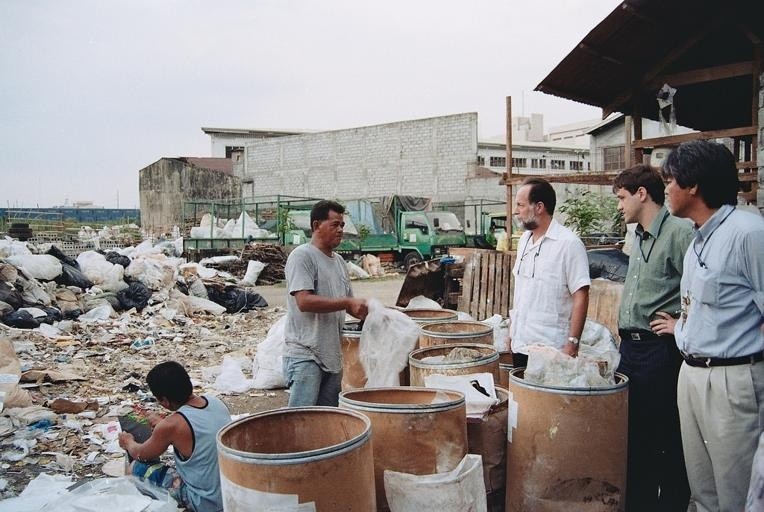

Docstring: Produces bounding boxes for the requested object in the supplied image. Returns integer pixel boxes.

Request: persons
[658,136,764,511]
[118,361,232,511]
[506,177,591,368]
[611,164,699,511]
[281,199,369,407]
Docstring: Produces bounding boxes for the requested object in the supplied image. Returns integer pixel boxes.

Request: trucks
[433,198,516,250]
[336,194,466,270]
[184,194,362,269]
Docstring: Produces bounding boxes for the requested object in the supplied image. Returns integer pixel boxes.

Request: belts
[685,352,764,367]
[619,330,661,341]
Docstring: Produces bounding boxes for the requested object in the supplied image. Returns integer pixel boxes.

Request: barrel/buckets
[505,367,629,512]
[212,406,378,510]
[338,388,469,512]
[334,306,621,388]
[461,390,509,511]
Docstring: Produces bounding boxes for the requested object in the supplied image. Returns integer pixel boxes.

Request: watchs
[568,337,580,345]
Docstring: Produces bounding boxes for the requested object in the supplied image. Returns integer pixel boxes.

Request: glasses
[518,253,538,278]
